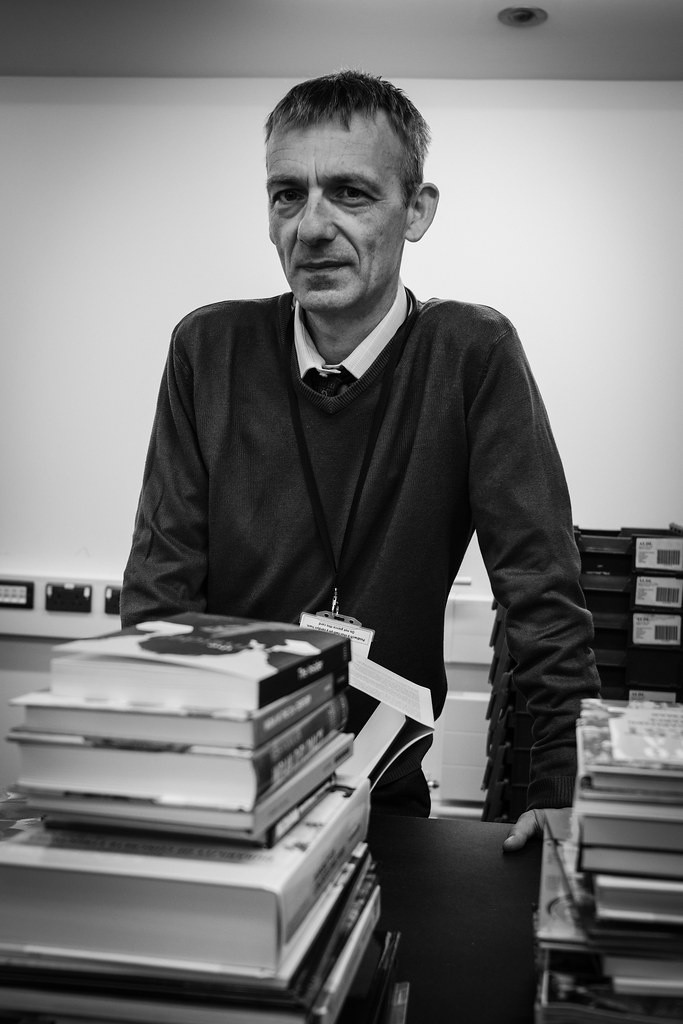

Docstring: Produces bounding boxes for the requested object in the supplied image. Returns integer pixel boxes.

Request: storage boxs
[481,524,683,824]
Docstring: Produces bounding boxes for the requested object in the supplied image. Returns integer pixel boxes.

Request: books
[535,698,682,1024]
[0,612,410,1024]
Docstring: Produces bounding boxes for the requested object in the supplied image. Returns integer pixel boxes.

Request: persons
[121,70,602,847]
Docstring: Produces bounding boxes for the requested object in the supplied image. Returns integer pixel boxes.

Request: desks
[367,814,542,1024]
